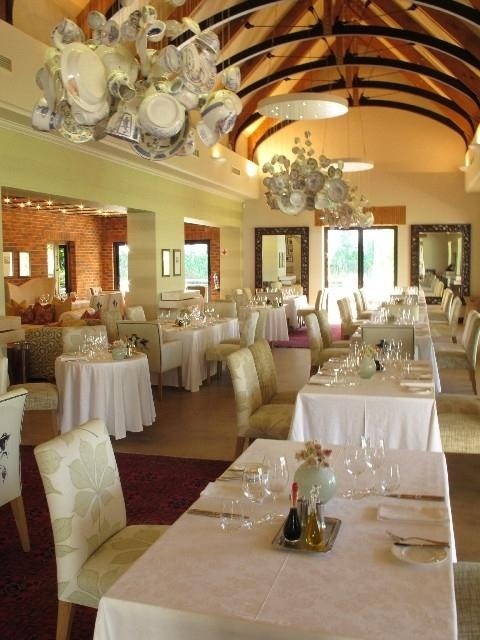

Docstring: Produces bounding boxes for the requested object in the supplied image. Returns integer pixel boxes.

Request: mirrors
[255,228,309,305]
[410,225,471,296]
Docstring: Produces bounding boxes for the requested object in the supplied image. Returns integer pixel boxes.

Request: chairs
[421,269,436,291]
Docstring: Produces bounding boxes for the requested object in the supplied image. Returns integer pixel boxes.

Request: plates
[276,168,349,216]
[392,542,448,569]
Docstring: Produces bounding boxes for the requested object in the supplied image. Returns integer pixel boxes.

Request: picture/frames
[19,250,32,278]
[161,249,171,276]
[173,249,181,276]
[3,250,15,279]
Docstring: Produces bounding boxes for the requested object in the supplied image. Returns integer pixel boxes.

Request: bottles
[284,483,302,542]
[305,485,323,544]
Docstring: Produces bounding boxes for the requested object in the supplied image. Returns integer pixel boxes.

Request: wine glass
[40,292,45,305]
[328,286,419,386]
[160,303,217,329]
[78,332,136,360]
[248,286,295,307]
[45,293,49,303]
[221,433,403,527]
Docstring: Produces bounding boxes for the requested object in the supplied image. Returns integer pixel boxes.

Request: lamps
[258,0,349,118]
[317,88,374,171]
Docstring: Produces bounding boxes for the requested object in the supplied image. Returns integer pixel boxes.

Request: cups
[30,11,241,150]
